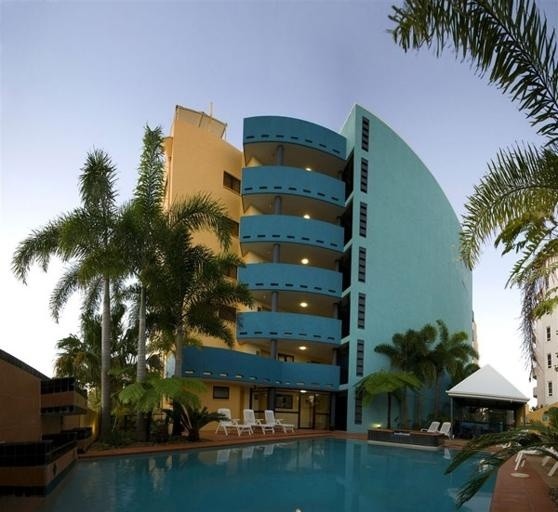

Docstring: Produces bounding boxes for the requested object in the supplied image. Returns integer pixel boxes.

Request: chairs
[514,447,558,477]
[438,422,453,440]
[420,422,441,432]
[265,410,295,434]
[242,409,276,436]
[215,408,251,436]
